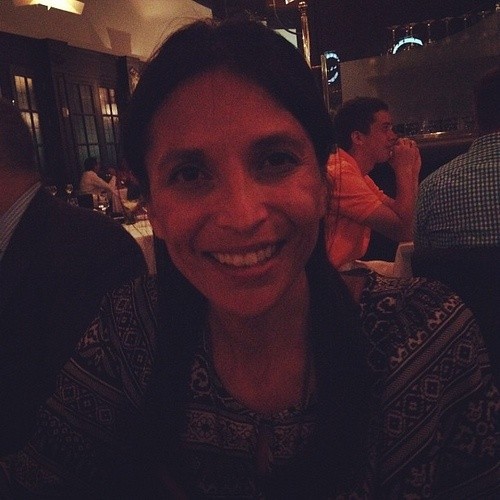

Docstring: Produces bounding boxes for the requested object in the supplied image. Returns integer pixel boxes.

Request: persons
[80,158,116,194]
[323,97,421,277]
[0,92,149,454]
[414,69,500,247]
[0,17,500,500]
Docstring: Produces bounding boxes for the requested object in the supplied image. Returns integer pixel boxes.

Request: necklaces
[206,347,312,459]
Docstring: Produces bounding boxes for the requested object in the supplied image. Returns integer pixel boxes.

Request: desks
[410,133,482,162]
